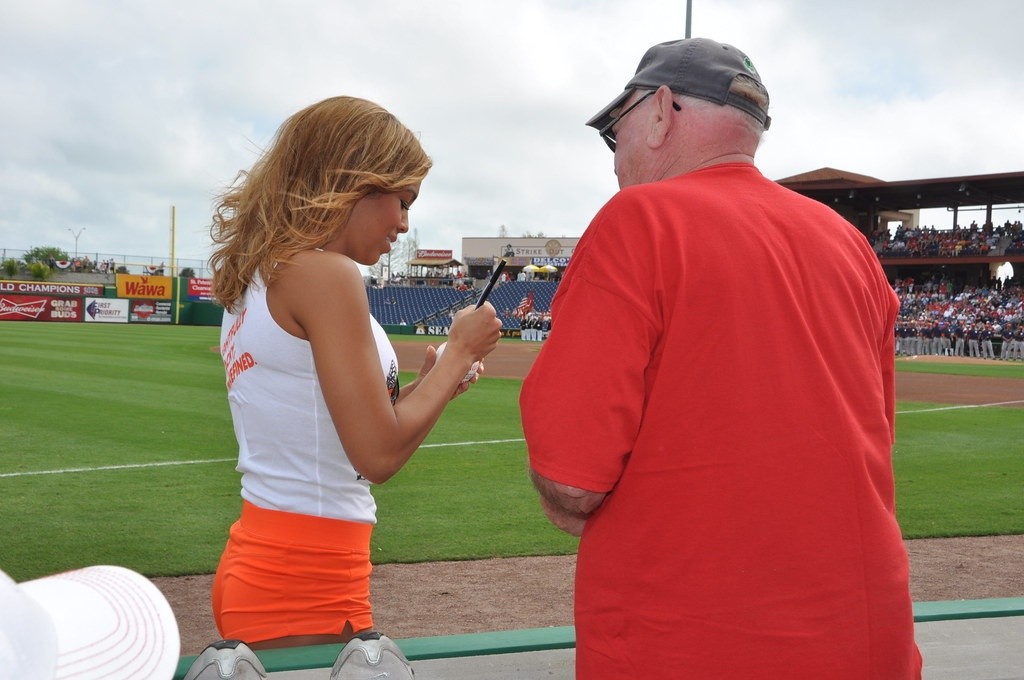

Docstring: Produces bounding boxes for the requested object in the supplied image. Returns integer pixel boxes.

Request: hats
[585,38,772,130]
[0,564,180,680]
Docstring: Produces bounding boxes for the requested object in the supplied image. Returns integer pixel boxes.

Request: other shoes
[184,639,266,680]
[331,631,414,680]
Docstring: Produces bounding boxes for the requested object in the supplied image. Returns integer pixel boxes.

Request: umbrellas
[522,264,538,279]
[539,264,558,280]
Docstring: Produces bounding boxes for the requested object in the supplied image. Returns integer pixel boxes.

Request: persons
[211,95,505,648]
[520,38,923,680]
[48,255,115,274]
[885,274,1024,362]
[501,271,526,282]
[520,315,552,341]
[859,219,1024,258]
[392,270,491,291]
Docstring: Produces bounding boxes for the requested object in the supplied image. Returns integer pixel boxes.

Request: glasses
[599,91,682,152]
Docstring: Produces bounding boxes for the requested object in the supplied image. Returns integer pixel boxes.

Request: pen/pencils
[476,258,507,310]
